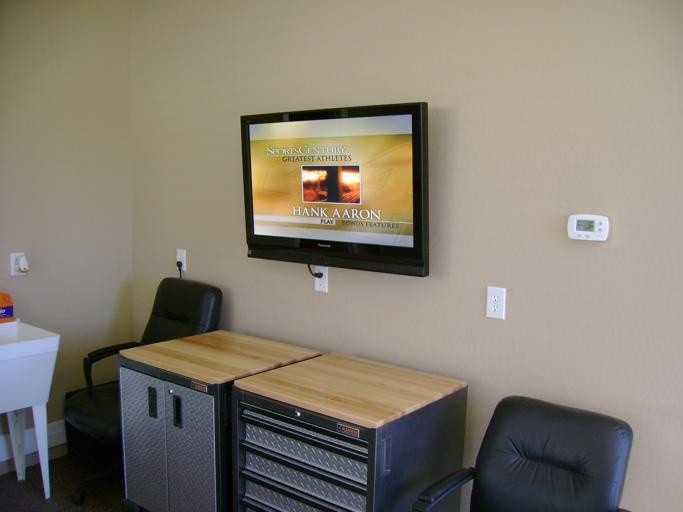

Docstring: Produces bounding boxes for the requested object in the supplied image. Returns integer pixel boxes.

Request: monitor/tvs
[240,101,429,277]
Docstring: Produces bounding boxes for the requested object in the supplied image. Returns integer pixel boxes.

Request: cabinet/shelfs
[228,349,469,512]
[117,324,325,512]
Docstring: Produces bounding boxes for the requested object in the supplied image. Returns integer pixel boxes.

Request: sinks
[0,322,60,414]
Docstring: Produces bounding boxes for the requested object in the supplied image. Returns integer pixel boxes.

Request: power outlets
[8,250,29,278]
[173,246,187,272]
[483,283,506,321]
[313,266,330,294]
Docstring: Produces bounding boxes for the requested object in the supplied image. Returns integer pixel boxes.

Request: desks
[0,319,65,502]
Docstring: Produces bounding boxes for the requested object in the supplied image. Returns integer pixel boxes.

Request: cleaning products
[0,290,17,323]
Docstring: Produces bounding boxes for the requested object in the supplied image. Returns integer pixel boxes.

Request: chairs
[61,274,228,505]
[410,392,635,512]
